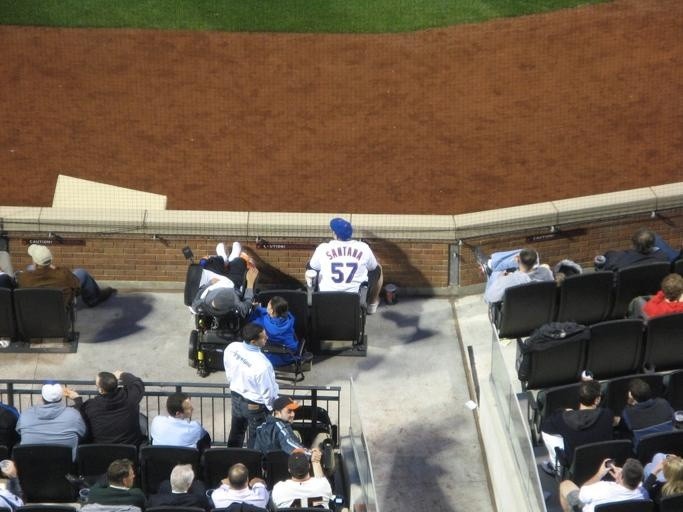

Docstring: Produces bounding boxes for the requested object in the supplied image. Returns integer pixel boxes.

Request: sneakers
[542,461,556,476]
[366,296,380,313]
[88,287,112,309]
[228,242,242,262]
[215,242,226,263]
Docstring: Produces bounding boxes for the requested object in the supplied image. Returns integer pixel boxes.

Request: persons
[0,265,22,290]
[557,458,648,512]
[641,452,682,503]
[474,245,552,304]
[539,380,614,476]
[304,218,384,315]
[597,228,670,288]
[617,380,675,456]
[247,295,298,368]
[190,240,258,320]
[625,273,682,330]
[0,371,335,512]
[15,240,114,308]
[222,322,279,449]
[653,233,683,274]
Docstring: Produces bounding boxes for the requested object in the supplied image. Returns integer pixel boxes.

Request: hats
[329,219,351,240]
[214,290,235,311]
[42,382,61,402]
[274,396,299,411]
[28,244,52,266]
[288,448,309,478]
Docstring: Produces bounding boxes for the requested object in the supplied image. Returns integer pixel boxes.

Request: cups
[384,284,398,304]
[79,489,91,502]
[674,411,683,428]
[594,256,606,272]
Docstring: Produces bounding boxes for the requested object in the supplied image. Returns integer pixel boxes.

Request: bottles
[196,351,206,377]
[305,270,317,287]
[581,369,594,382]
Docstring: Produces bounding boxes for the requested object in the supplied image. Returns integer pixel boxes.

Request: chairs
[255,288,367,381]
[0,287,81,354]
